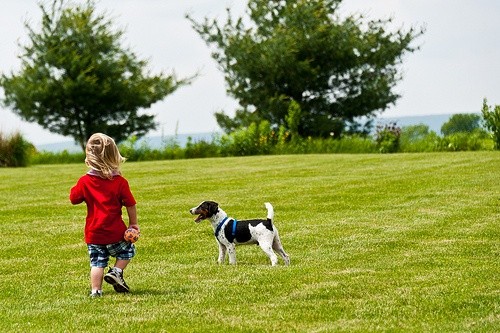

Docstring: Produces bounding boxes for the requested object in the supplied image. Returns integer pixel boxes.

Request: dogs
[189,200,290,267]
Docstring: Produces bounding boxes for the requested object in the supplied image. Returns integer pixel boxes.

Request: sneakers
[104,267,129,293]
[90,290,103,298]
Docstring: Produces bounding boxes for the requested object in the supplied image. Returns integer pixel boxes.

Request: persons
[70,133,140,297]
